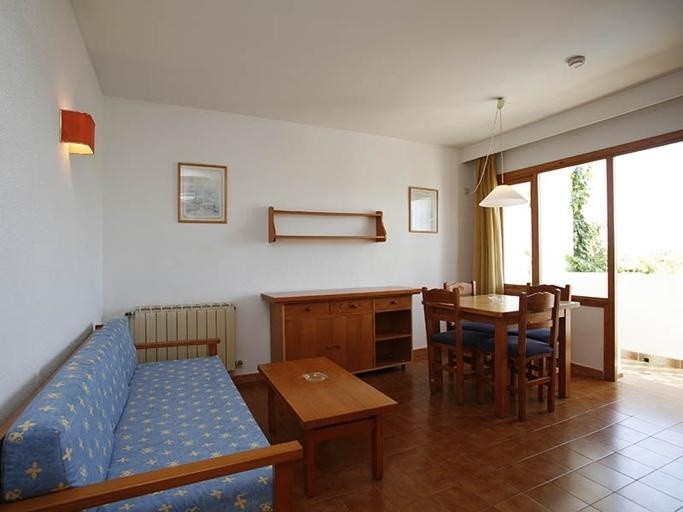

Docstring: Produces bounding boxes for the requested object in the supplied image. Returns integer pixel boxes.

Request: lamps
[61,109,95,154]
[479,99,528,205]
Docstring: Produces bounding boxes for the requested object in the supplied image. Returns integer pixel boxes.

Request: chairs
[421,281,581,423]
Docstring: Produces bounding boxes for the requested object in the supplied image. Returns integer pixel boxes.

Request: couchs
[0,319,304,512]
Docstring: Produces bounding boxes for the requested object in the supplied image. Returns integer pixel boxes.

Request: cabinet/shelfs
[269,206,387,242]
[270,295,412,374]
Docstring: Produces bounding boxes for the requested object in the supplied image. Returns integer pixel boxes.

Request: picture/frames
[409,187,438,233]
[177,162,227,223]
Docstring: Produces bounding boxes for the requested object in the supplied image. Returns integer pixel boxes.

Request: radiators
[125,303,242,372]
